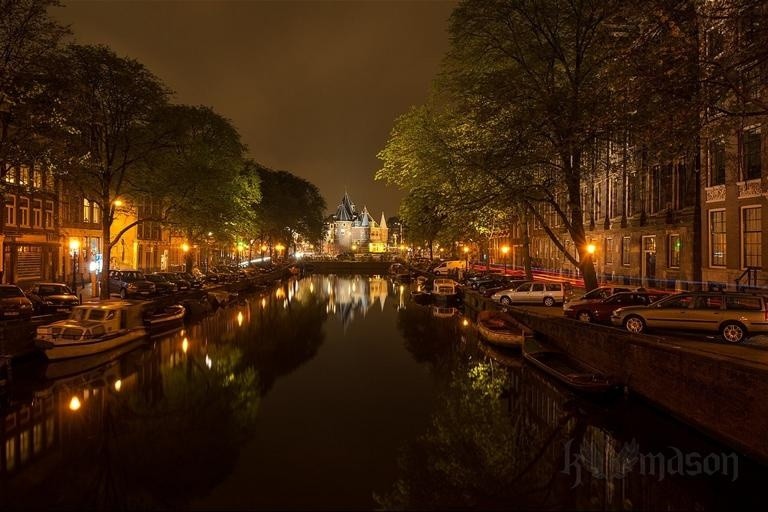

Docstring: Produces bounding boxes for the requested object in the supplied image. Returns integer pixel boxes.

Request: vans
[492,281,565,307]
[564,285,649,304]
[611,292,767,344]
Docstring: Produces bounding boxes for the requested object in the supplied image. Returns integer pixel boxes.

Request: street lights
[502,246,509,273]
[393,222,403,245]
[464,247,469,272]
[70,240,80,294]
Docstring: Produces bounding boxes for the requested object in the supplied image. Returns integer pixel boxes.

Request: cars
[409,247,466,276]
[1,284,34,320]
[109,262,277,298]
[27,282,80,313]
[463,270,533,297]
[564,292,674,324]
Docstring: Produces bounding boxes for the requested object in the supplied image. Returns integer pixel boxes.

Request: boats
[477,309,536,348]
[521,328,629,397]
[288,260,317,279]
[187,287,238,317]
[33,300,145,360]
[148,321,184,340]
[429,302,463,322]
[145,303,186,328]
[389,263,430,291]
[35,338,145,392]
[410,289,431,304]
[475,338,523,371]
[430,278,460,305]
